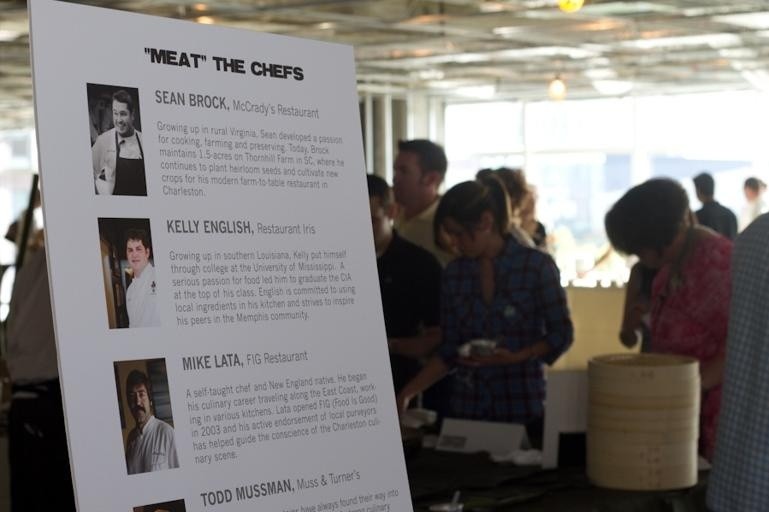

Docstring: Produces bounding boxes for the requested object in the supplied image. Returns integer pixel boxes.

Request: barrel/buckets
[587,354,700,490]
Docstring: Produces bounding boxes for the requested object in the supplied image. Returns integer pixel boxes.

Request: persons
[394,168,573,451]
[604,173,769,512]
[92,90,147,196]
[392,139,462,270]
[367,173,447,394]
[125,229,159,328]
[499,167,556,250]
[126,369,180,475]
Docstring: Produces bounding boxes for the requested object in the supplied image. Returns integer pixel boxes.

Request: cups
[429,502,465,512]
[470,338,497,357]
